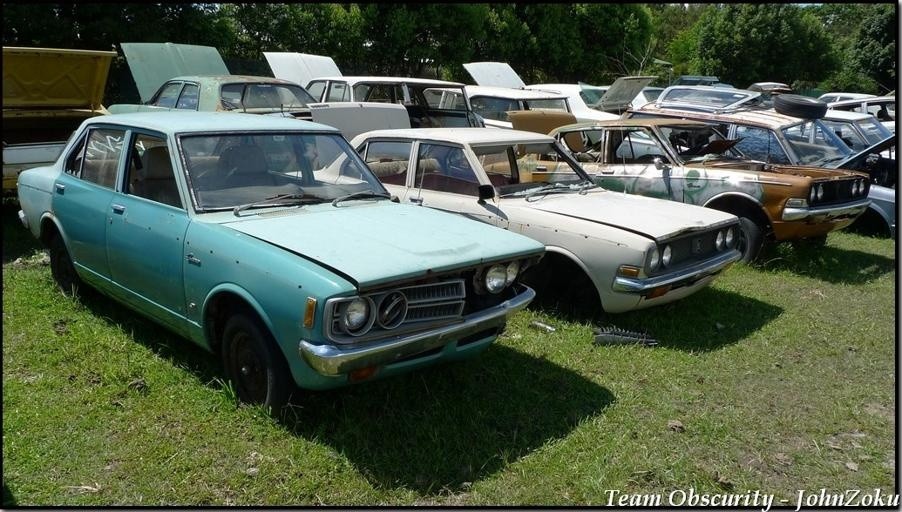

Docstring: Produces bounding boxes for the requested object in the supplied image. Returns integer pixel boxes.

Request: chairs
[370,159,444,187]
[134,143,271,211]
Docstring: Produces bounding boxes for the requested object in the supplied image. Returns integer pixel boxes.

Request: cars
[1,43,895,410]
